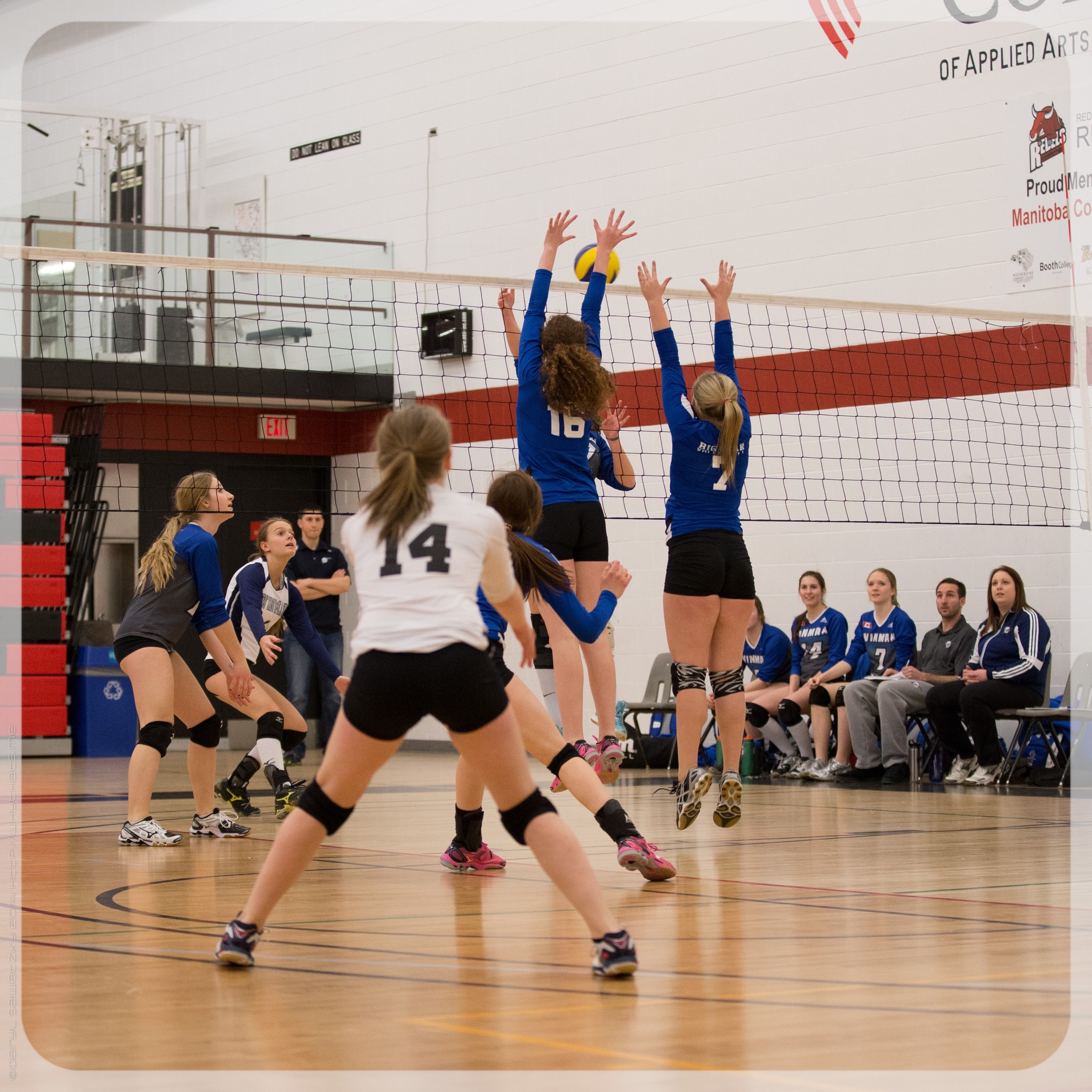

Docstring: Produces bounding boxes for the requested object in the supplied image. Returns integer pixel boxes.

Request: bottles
[753,737,764,775]
[740,735,754,776]
[929,740,943,781]
[908,739,923,782]
[716,735,724,766]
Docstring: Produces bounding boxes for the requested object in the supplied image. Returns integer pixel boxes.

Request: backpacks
[1020,694,1086,788]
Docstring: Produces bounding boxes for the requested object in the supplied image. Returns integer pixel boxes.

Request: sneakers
[282,753,301,767]
[439,841,506,871]
[964,757,1010,786]
[945,754,979,784]
[275,779,311,819]
[653,767,713,830]
[713,769,743,828]
[550,740,600,793]
[213,919,270,966]
[617,835,676,881]
[774,753,802,777]
[189,808,251,838]
[811,757,853,781]
[591,930,638,977]
[799,758,829,777]
[215,777,261,816]
[791,756,815,777]
[592,735,624,784]
[118,816,183,846]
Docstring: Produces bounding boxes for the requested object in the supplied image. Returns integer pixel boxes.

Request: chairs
[997,654,1052,785]
[911,709,970,784]
[624,654,677,770]
[1005,653,1092,787]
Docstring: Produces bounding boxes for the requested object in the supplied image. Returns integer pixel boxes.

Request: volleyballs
[573,242,620,284]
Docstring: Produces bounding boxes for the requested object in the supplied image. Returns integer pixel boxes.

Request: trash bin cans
[68,644,138,757]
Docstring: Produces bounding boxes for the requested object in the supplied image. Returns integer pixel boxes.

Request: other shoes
[836,763,884,782]
[882,762,910,785]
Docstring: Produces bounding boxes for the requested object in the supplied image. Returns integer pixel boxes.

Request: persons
[497,208,758,830]
[214,404,638,977]
[112,472,255,846]
[282,503,351,766]
[441,470,676,882]
[201,517,351,821]
[705,562,1052,787]
